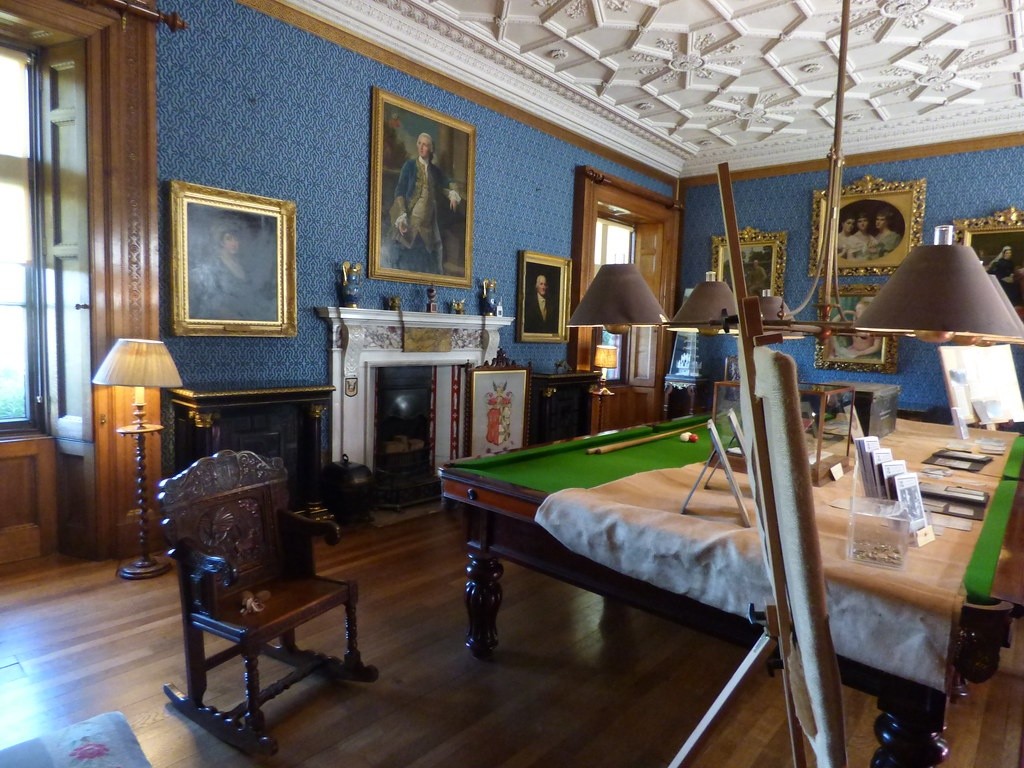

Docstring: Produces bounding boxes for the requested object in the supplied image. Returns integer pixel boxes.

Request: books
[855,436,926,531]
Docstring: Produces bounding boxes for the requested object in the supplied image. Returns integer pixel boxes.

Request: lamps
[95,337,186,579]
[567,1,1024,348]
[588,343,621,432]
[743,293,806,340]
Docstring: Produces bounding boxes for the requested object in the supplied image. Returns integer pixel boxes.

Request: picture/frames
[953,206,1024,322]
[710,225,788,314]
[516,249,574,343]
[810,283,899,375]
[168,181,303,336]
[807,174,927,278]
[371,84,475,288]
[463,365,531,456]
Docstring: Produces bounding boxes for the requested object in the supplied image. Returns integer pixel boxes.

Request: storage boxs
[709,378,856,488]
[846,499,910,573]
[801,380,905,439]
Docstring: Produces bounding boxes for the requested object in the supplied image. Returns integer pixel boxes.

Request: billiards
[680,430,698,443]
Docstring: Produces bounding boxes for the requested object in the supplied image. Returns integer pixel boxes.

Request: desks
[436,399,1024,768]
[661,375,711,419]
[531,366,600,444]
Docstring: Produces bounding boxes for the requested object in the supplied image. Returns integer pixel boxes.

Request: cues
[585,421,711,456]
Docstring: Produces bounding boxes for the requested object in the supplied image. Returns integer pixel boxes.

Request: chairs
[154,450,379,757]
